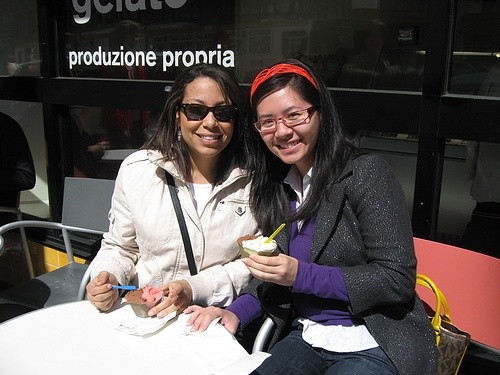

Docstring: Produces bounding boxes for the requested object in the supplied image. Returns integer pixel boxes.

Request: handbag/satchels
[416,272,471,375]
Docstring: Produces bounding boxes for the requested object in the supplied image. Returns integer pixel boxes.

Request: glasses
[178,102,238,123]
[253,105,319,133]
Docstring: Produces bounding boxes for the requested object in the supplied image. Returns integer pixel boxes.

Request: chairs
[250,236,500,375]
[0,220,107,310]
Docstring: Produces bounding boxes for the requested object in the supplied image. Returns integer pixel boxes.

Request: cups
[236,235,278,260]
[126,291,163,318]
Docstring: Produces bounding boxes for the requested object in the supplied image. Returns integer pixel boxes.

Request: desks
[0,297,250,375]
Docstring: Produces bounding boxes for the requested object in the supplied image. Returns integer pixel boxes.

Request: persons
[182,59,436,375]
[85,61,253,319]
[0,111,36,224]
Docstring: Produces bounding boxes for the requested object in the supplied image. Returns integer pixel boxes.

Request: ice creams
[238,235,278,259]
[125,284,163,318]
